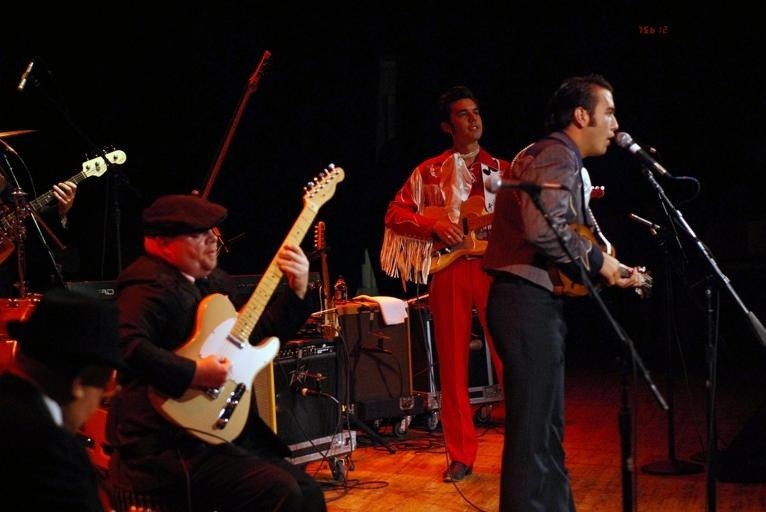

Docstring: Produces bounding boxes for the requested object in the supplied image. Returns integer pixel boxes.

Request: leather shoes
[444,460,469,484]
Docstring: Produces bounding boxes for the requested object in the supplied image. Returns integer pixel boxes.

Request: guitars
[552,223,653,298]
[147,163,345,445]
[313,221,331,311]
[412,195,495,273]
[0,145,126,264]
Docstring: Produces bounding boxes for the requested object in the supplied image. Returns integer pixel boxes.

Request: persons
[0,167,78,296]
[470,73,655,512]
[379,86,512,485]
[112,195,325,512]
[1,289,128,512]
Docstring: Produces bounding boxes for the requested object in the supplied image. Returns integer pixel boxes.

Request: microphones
[485,177,564,194]
[302,387,342,406]
[615,132,672,179]
[17,59,35,91]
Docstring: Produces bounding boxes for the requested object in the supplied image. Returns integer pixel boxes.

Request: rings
[447,234,450,237]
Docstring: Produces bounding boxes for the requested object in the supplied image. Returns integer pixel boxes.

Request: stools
[102,454,189,512]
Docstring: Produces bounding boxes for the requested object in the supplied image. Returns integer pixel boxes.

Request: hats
[141,193,226,236]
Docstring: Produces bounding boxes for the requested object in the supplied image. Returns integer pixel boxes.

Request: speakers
[337,300,409,419]
[253,347,358,465]
